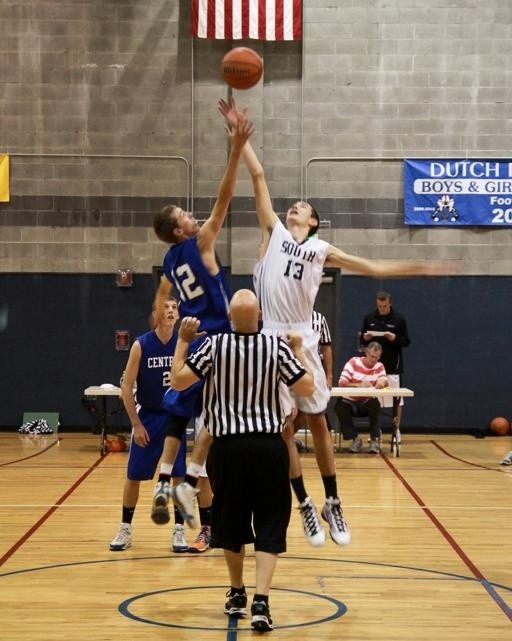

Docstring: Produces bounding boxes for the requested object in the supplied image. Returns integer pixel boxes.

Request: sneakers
[188,525,212,553]
[151,481,169,524]
[224,586,247,615]
[348,438,362,453]
[321,496,350,545]
[393,430,401,443]
[171,481,200,528]
[368,439,381,454]
[297,496,326,547]
[172,523,188,553]
[109,523,133,551]
[251,601,273,630]
[500,452,512,464]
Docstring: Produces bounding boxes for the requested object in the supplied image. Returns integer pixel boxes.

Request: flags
[189,0,305,45]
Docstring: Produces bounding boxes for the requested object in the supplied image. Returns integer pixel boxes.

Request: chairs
[335,377,383,454]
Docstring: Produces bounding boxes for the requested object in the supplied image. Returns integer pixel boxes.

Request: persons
[358,290,411,445]
[151,116,257,530]
[164,284,320,636]
[333,343,388,457]
[107,294,335,555]
[216,96,462,548]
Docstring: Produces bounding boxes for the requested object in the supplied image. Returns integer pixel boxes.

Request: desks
[82,383,416,459]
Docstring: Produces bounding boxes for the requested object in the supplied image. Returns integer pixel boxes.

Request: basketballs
[491,416,510,437]
[222,46,263,90]
